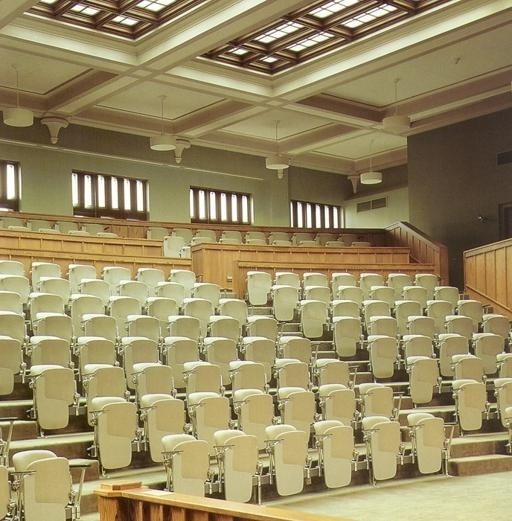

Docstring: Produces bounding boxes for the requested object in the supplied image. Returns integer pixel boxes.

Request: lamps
[379,76,412,134]
[356,137,385,188]
[262,120,291,176]
[2,61,34,129]
[151,94,175,152]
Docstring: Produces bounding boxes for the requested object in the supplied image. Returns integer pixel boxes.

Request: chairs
[0,218,371,246]
[0,260,512,519]
